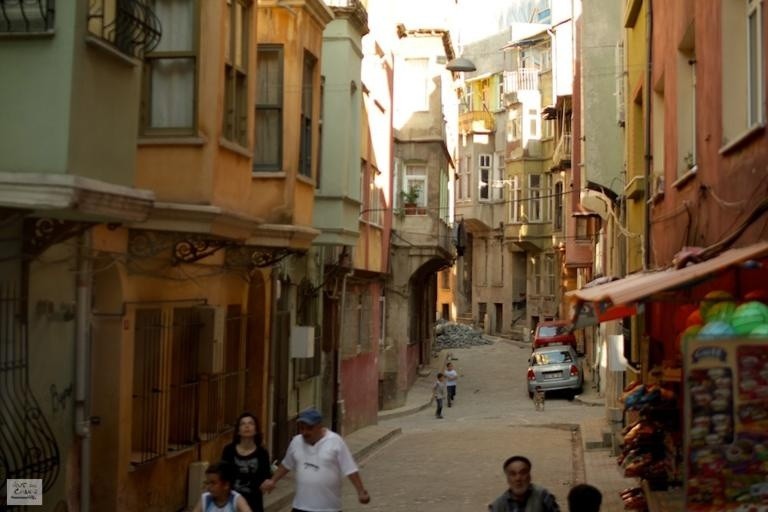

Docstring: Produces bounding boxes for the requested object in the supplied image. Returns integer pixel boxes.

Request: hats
[296,410,320,426]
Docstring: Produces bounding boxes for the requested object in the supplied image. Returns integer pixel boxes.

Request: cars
[527,345,584,401]
[531,320,577,352]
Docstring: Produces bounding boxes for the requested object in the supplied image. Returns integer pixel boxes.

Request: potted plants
[402,184,421,214]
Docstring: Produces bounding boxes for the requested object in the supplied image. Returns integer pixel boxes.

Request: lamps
[446,49,475,72]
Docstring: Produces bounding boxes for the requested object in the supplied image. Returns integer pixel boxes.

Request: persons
[271,407,368,512]
[443,363,458,407]
[562,482,606,512]
[532,384,546,412]
[433,373,446,418]
[190,464,250,512]
[226,413,271,501]
[481,456,558,511]
